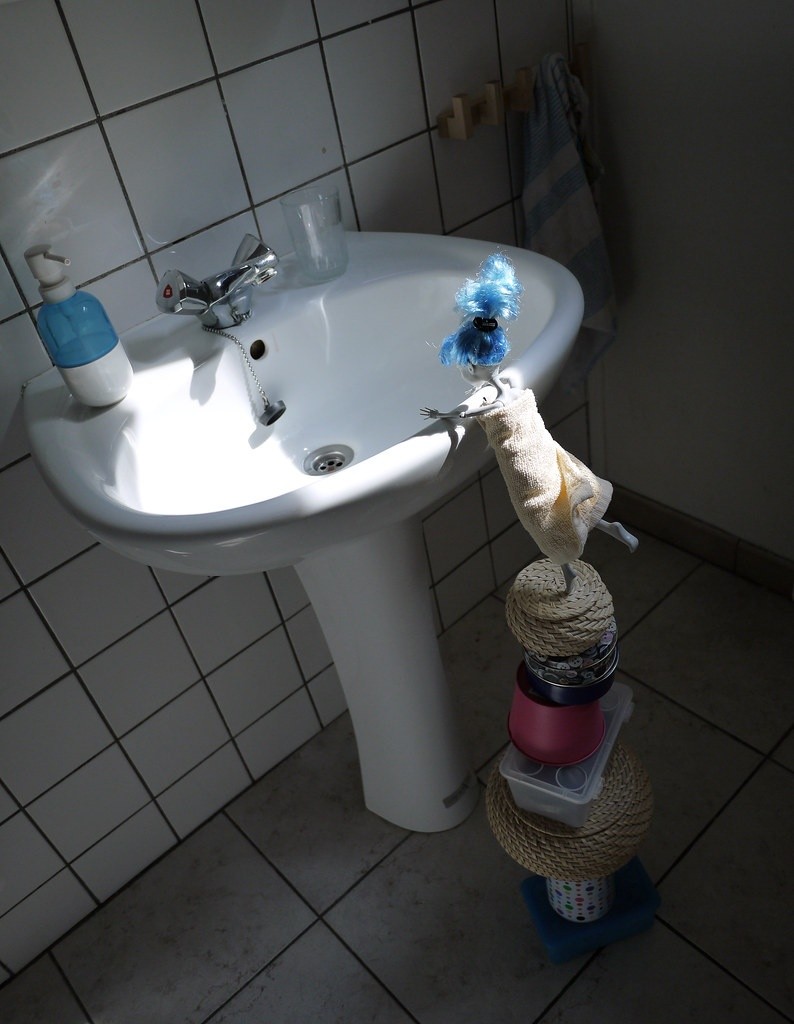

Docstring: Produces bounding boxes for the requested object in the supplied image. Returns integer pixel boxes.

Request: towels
[516,45,636,388]
[475,386,613,564]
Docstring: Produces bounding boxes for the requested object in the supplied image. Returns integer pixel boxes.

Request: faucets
[148,234,278,335]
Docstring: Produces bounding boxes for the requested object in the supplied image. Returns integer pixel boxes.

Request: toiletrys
[22,244,134,407]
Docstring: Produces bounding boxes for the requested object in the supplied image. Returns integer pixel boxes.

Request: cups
[279,185,349,282]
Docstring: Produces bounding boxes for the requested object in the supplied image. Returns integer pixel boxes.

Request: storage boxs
[520,855,662,962]
[498,683,635,826]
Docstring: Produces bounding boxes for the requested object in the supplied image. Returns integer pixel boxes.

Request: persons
[419,246,639,595]
